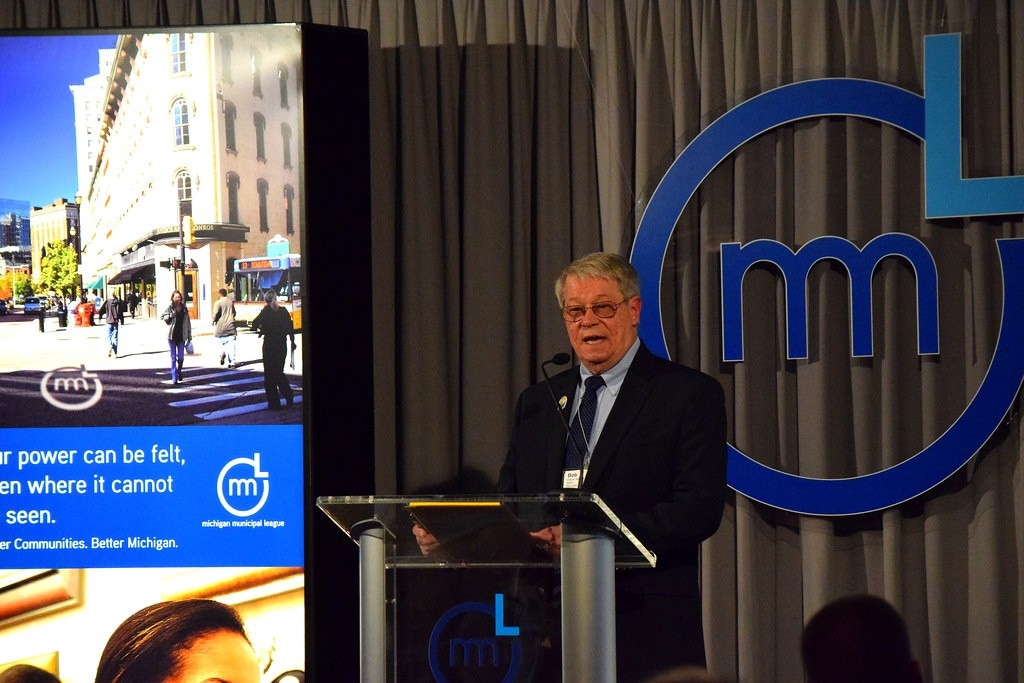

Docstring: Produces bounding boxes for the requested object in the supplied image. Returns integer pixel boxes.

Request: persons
[251,289,296,409]
[212,288,238,367]
[279,281,294,294]
[126,290,138,319]
[161,290,191,385]
[801,594,925,683]
[97,290,124,357]
[413,252,726,683]
[94,598,261,683]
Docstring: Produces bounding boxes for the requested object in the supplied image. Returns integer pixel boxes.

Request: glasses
[560,298,633,323]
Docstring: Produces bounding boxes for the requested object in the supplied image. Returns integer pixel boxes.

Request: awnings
[84,276,103,289]
[108,264,155,284]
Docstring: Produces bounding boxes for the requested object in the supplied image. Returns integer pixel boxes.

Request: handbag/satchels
[184,338,196,354]
[165,305,175,325]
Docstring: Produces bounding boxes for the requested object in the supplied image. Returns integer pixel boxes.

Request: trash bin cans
[59,309,66,327]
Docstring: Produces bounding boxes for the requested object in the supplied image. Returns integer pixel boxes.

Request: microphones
[540,353,586,494]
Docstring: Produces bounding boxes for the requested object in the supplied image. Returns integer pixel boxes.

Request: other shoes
[115,354,118,358]
[268,404,282,411]
[220,353,226,365]
[228,364,236,369]
[173,381,177,384]
[286,399,294,407]
[178,375,182,382]
[108,347,112,357]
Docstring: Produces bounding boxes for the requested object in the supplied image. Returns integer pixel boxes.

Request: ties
[562,376,607,473]
